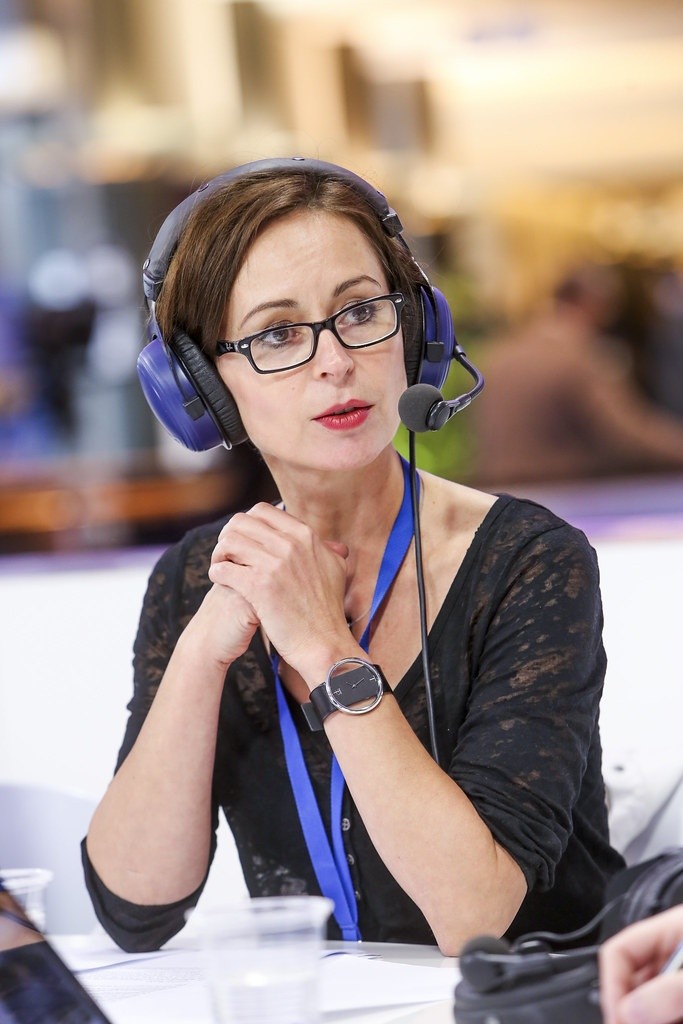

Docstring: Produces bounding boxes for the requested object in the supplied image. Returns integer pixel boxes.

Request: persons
[473,270,683,484]
[78,158,622,958]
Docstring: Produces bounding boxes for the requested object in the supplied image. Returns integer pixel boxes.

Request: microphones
[398,339,488,433]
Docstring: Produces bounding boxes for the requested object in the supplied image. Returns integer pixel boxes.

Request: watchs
[301,657,392,732]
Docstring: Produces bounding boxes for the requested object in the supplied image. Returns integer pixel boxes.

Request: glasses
[216,292,407,375]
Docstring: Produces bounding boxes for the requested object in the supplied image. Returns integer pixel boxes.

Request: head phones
[137,156,458,455]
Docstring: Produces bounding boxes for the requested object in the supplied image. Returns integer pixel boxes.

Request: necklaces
[347,476,425,627]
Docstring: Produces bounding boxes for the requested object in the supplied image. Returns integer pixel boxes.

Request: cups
[185,896,334,1024]
[0,870,55,932]
